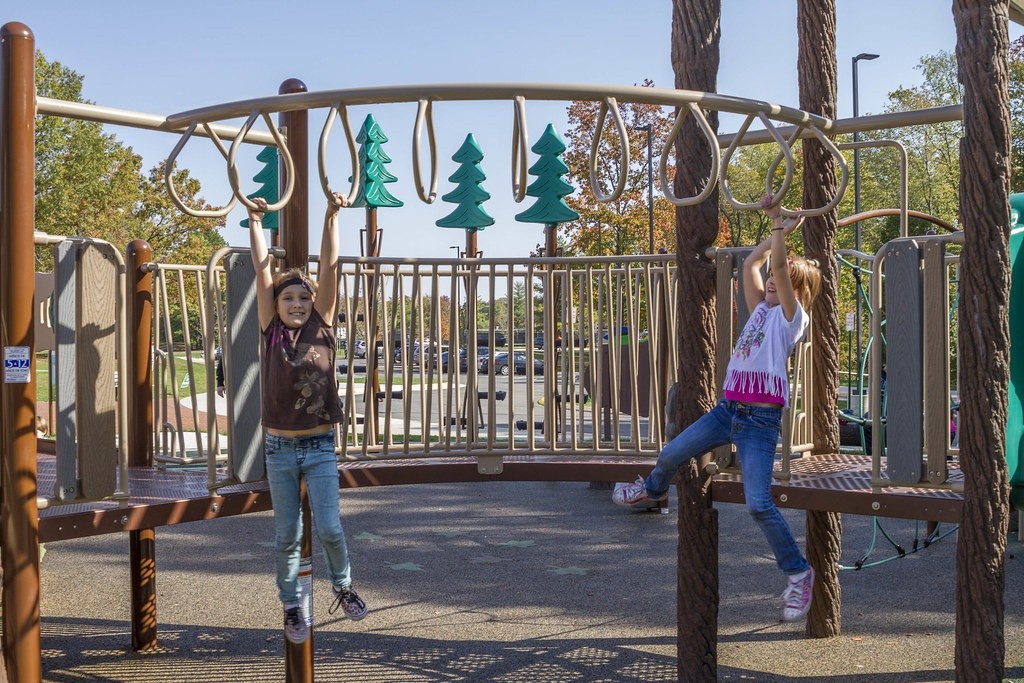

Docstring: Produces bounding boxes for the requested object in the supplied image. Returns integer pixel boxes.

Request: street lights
[450,246,461,342]
[850,52,882,395]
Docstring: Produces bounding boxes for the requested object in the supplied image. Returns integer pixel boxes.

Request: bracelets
[248,216,262,223]
[770,227,783,233]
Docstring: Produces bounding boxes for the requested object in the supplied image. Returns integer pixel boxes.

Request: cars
[339,329,589,376]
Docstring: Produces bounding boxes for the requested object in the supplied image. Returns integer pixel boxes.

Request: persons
[612,196,822,621]
[216,356,224,397]
[245,191,367,645]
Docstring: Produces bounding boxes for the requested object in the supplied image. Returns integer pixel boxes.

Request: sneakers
[612,474,667,506]
[328,584,367,620]
[780,566,815,621]
[284,604,307,643]
